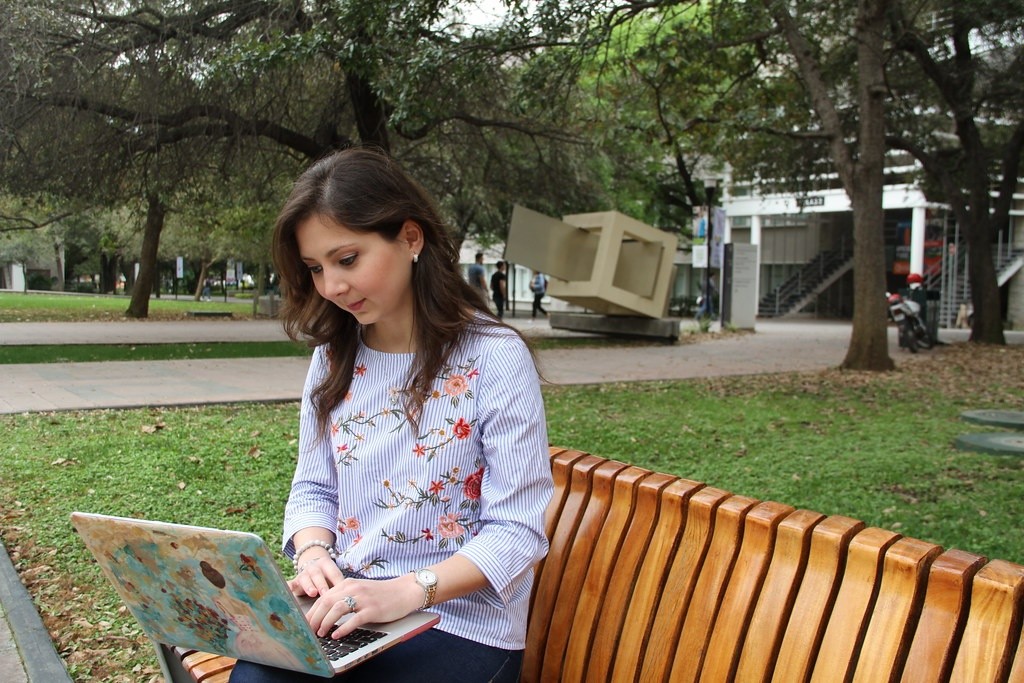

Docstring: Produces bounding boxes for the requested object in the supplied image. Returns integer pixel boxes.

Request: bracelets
[293,541,336,575]
[299,558,320,574]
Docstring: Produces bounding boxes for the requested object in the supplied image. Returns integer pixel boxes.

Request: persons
[201,278,211,301]
[528,272,550,323]
[490,261,506,320]
[227,151,554,683]
[469,253,489,306]
[695,296,713,319]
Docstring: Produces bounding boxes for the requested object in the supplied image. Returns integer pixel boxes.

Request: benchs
[155,439,1024,683]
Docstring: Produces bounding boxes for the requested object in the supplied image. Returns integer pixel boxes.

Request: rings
[344,596,356,611]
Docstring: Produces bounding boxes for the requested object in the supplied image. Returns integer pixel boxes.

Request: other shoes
[545,313,550,321]
[528,318,537,323]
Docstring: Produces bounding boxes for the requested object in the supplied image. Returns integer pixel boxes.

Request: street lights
[702,170,723,332]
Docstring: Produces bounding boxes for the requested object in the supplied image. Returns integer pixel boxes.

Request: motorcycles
[885,274,932,353]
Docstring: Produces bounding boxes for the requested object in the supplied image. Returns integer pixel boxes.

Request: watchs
[410,569,438,612]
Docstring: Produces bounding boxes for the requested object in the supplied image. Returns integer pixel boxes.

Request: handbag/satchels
[544,280,548,290]
[696,297,704,305]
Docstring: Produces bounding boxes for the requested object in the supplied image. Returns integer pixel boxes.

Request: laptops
[69,512,441,679]
